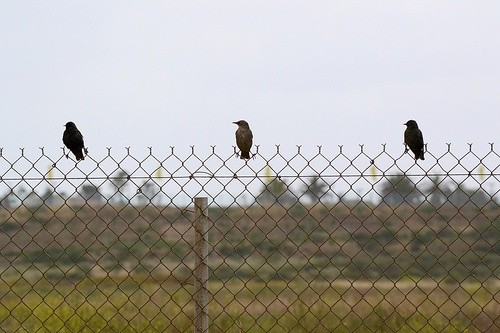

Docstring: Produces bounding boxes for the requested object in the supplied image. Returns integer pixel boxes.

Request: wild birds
[232,119,255,161]
[402,119,425,161]
[62,121,87,162]
